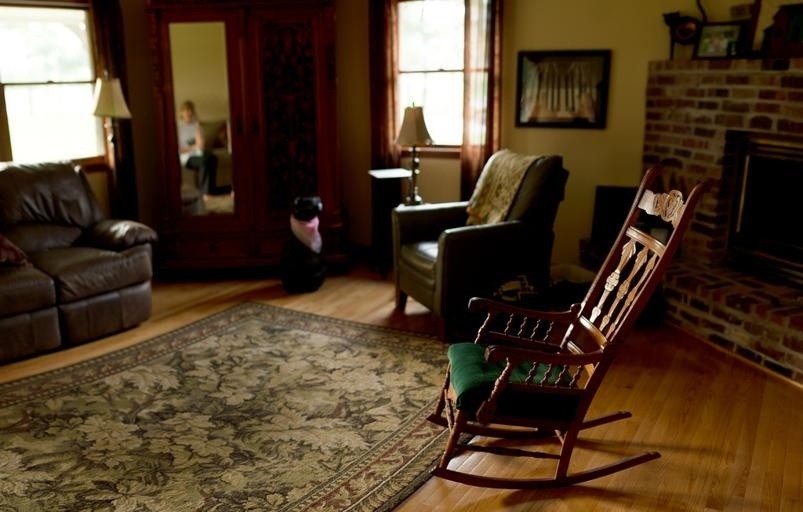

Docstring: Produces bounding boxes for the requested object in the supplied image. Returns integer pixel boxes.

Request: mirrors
[168,22,234,215]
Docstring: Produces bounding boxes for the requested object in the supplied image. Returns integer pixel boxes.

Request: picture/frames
[513,48,611,131]
[688,19,746,60]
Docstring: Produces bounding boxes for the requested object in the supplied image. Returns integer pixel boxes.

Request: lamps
[87,68,134,220]
[393,101,434,207]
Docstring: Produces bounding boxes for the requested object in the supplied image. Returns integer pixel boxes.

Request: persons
[175,101,220,198]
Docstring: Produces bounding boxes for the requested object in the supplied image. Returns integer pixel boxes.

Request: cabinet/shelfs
[144,7,253,239]
[245,8,334,223]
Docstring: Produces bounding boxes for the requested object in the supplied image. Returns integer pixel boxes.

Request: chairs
[384,147,571,340]
[424,167,703,491]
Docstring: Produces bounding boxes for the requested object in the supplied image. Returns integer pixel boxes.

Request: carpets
[0,298,492,512]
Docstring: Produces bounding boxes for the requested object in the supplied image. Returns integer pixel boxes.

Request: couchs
[178,116,233,196]
[0,160,158,367]
